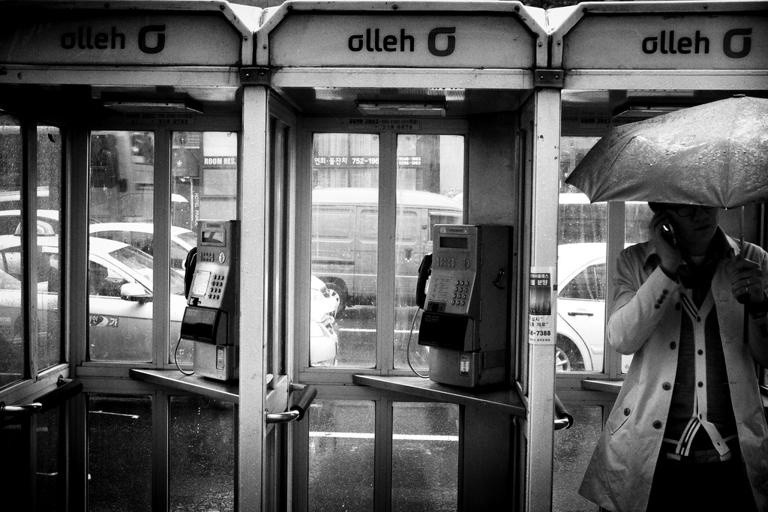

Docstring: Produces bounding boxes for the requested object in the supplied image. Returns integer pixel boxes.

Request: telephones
[415,224,514,353]
[181,217,241,347]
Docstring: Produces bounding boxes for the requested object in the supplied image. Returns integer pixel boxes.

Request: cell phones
[659,218,680,247]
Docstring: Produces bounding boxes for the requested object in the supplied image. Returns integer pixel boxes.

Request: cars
[1,234,341,400]
[86,221,341,319]
[554,190,655,379]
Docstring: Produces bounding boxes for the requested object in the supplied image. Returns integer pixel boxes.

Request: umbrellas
[566,97,768,356]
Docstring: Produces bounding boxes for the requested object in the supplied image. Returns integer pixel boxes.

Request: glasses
[673,204,715,217]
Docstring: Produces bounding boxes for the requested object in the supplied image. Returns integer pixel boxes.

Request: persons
[579,203,768,512]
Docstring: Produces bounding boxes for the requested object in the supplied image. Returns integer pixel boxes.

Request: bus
[0,121,202,237]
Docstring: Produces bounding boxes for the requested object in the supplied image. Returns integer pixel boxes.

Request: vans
[309,184,465,319]
